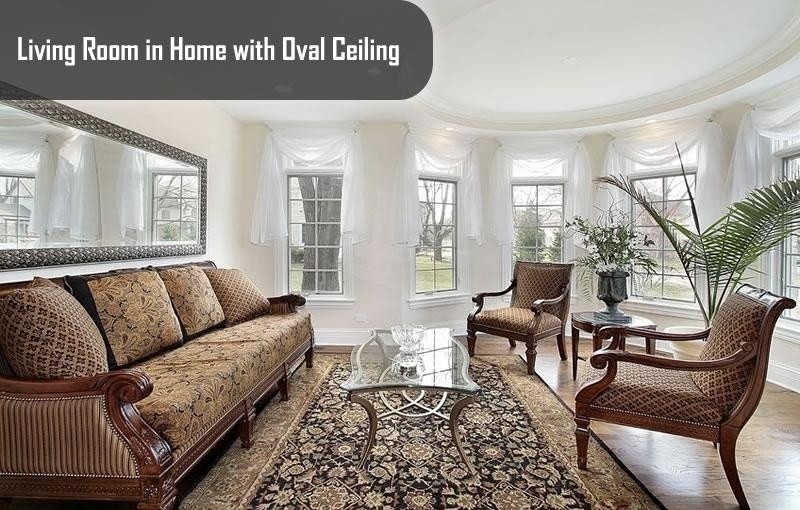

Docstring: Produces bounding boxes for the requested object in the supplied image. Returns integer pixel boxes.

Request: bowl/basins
[390,324,426,352]
[390,350,428,380]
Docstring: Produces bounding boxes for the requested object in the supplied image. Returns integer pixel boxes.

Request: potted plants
[590,141,800,360]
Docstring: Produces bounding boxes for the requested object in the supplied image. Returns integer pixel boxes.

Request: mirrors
[0,80,207,270]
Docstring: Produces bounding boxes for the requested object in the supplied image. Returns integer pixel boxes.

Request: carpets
[169,354,667,509]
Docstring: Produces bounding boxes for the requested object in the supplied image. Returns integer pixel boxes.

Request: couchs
[0,259,314,510]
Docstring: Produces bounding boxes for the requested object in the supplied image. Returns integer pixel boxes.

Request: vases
[593,271,632,324]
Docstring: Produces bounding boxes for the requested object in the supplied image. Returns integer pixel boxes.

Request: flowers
[553,184,662,302]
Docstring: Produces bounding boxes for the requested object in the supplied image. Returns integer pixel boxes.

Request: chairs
[465,260,575,375]
[573,283,796,509]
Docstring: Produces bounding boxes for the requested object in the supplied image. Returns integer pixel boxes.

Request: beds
[339,328,481,476]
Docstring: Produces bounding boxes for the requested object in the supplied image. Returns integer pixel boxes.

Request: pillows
[0,275,109,382]
[202,266,273,328]
[155,265,225,341]
[64,264,183,371]
